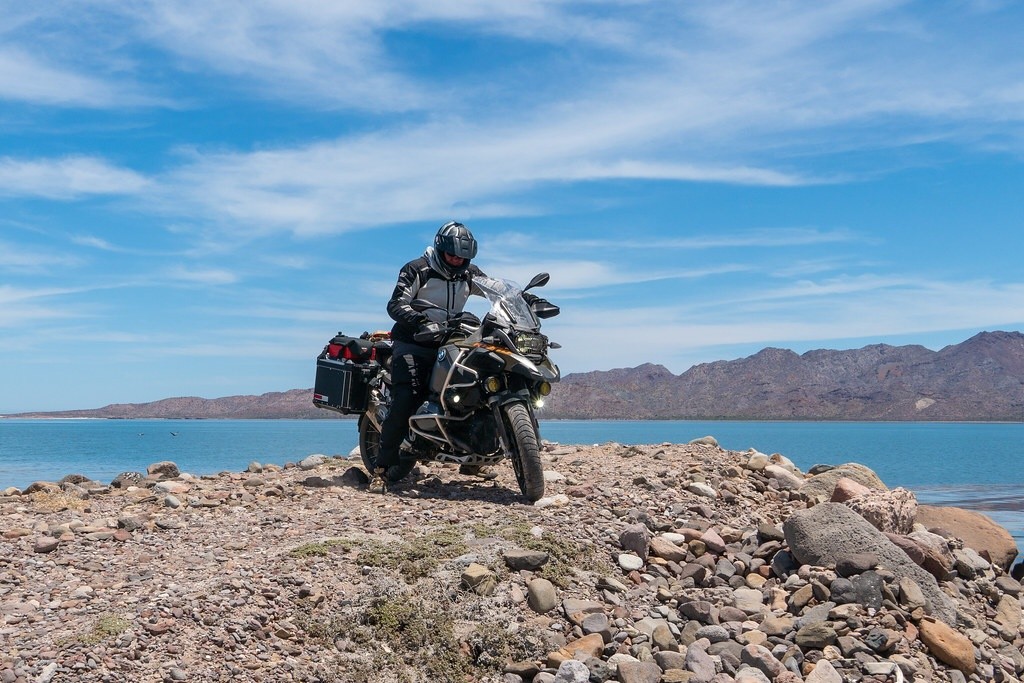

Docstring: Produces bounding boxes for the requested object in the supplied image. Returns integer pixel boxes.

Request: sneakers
[367,473,387,493]
[459,465,498,478]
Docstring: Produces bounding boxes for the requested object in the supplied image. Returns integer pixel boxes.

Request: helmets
[434,220,478,274]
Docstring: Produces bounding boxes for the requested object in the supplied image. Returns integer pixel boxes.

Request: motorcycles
[312,273,562,501]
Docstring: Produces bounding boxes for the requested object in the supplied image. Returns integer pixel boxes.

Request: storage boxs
[311,358,370,413]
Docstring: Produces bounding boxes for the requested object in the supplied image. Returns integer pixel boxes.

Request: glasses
[446,252,465,259]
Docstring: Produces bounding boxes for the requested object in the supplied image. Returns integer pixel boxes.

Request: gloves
[416,317,433,331]
[529,294,547,306]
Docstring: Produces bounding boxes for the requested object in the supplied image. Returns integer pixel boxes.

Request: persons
[368,222,548,493]
